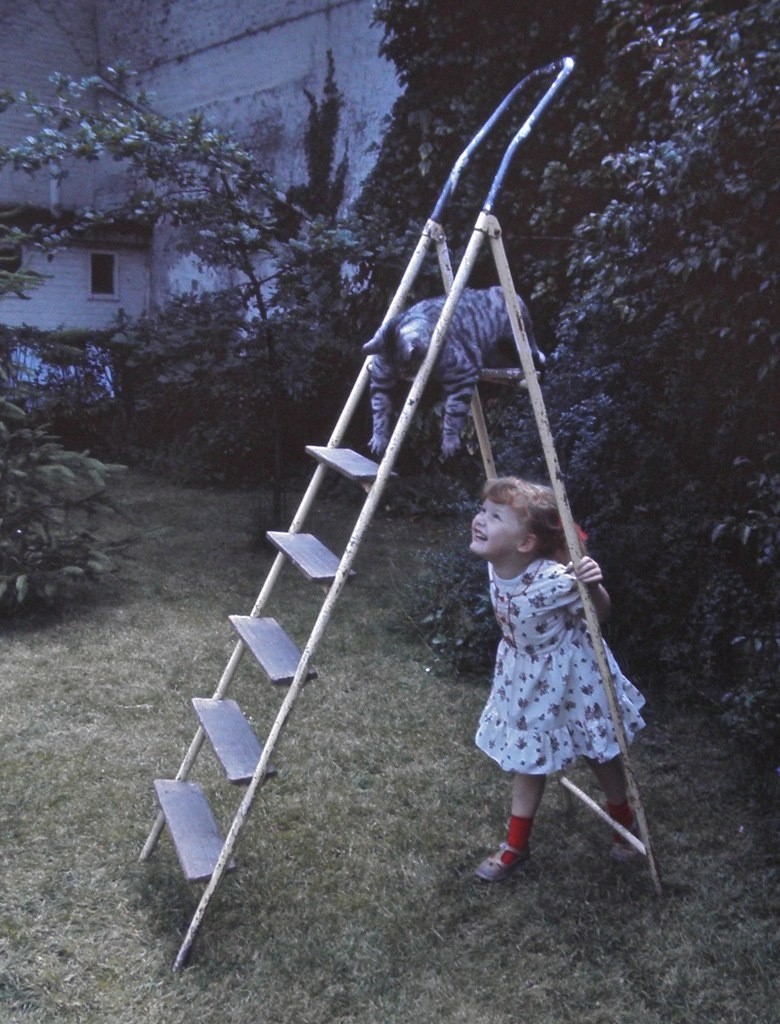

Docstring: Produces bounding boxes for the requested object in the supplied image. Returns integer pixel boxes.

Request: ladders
[135,57,661,971]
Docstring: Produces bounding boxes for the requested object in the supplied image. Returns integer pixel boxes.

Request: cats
[362,286,547,459]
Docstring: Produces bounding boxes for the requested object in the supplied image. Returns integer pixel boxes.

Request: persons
[464,472,646,879]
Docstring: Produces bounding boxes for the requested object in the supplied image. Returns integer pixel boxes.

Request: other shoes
[475,843,532,881]
[609,826,641,863]
